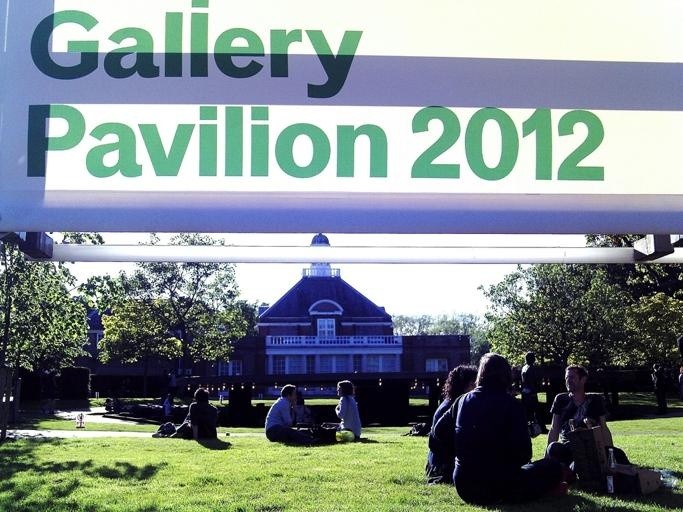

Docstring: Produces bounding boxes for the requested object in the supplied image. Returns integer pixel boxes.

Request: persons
[323,379,361,442]
[169,387,219,438]
[515,352,550,435]
[423,364,477,486]
[543,362,614,489]
[649,361,667,415]
[219,389,224,404]
[431,349,571,507]
[674,366,682,401]
[161,394,173,422]
[291,390,311,425]
[264,383,314,446]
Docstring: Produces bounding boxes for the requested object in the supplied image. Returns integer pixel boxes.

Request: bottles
[606,448,619,499]
